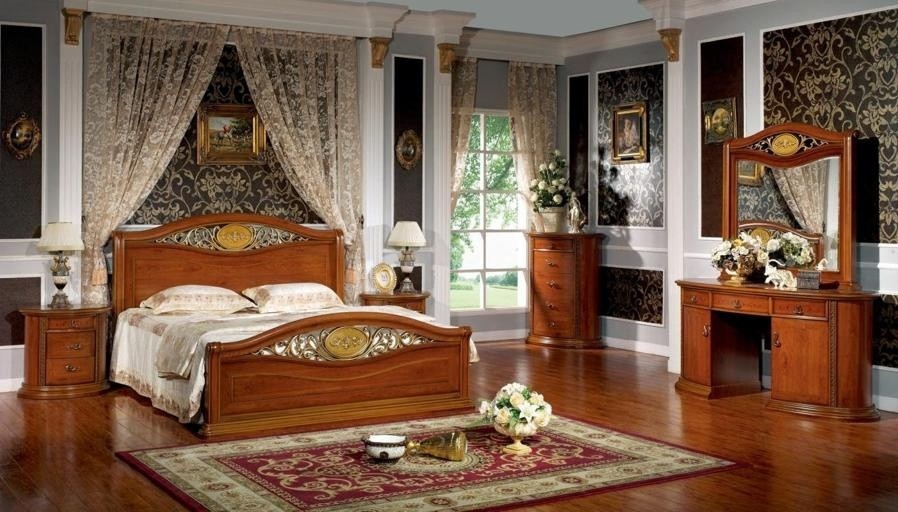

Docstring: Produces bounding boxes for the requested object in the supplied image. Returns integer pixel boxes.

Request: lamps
[387,221,426,296]
[37,222,85,308]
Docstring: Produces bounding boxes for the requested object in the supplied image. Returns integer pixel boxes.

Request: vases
[725,268,752,285]
[494,422,533,455]
[538,208,567,232]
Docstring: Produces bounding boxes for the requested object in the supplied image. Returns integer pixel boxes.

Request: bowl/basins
[361,434,410,463]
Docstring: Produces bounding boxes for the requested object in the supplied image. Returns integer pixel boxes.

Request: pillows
[140,285,258,314]
[242,282,347,314]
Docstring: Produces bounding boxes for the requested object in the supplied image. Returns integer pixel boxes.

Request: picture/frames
[703,97,738,145]
[737,159,763,187]
[197,103,267,166]
[611,101,648,165]
[2,112,41,162]
[395,127,424,171]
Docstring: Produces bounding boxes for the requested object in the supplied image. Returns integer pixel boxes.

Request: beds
[738,220,824,270]
[108,213,480,442]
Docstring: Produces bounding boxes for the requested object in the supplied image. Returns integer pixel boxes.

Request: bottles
[407,431,466,461]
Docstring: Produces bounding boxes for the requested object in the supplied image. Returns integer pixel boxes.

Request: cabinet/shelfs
[675,279,882,423]
[525,232,606,350]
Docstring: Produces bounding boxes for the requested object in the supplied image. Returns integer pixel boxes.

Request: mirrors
[723,123,860,287]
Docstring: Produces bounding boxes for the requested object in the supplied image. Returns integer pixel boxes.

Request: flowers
[711,232,770,274]
[478,383,552,437]
[530,149,571,207]
[767,232,815,268]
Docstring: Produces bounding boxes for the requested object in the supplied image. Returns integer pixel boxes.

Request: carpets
[115,411,761,511]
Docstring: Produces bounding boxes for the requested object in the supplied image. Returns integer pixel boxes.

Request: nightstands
[18,305,112,400]
[359,291,430,314]
